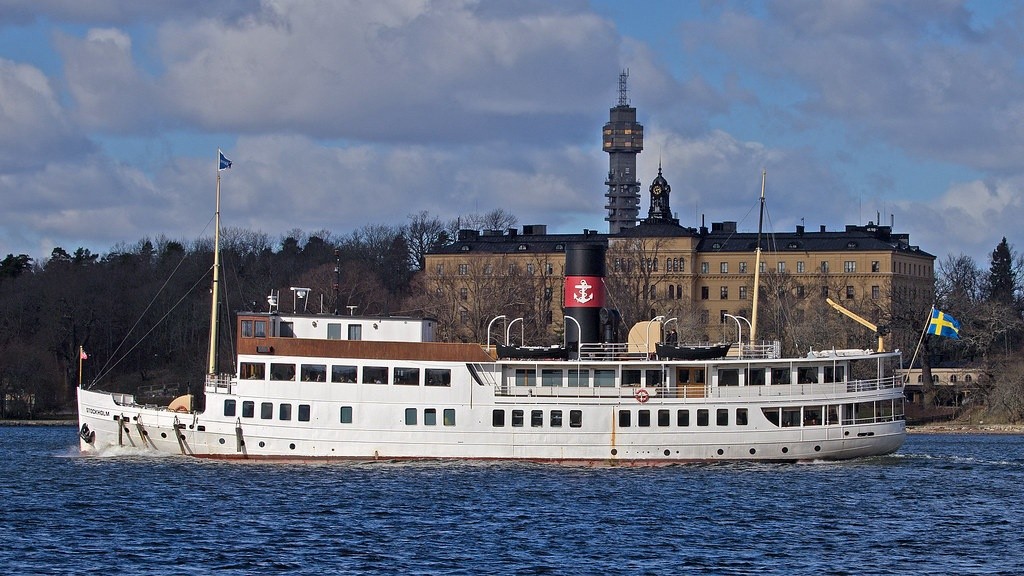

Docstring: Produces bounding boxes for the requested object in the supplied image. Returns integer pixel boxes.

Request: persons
[248,369,451,386]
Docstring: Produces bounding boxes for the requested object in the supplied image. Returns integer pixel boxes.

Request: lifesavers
[635,389,649,403]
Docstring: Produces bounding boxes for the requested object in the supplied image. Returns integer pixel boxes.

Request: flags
[218,150,233,171]
[80,348,87,359]
[928,310,960,340]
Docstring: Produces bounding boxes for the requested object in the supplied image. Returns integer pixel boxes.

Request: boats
[77,146,907,466]
[495,341,570,359]
[655,341,732,359]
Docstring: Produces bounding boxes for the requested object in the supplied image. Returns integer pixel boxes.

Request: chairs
[655,343,732,362]
[495,343,569,361]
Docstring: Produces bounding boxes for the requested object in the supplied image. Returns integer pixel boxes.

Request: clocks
[652,185,662,195]
